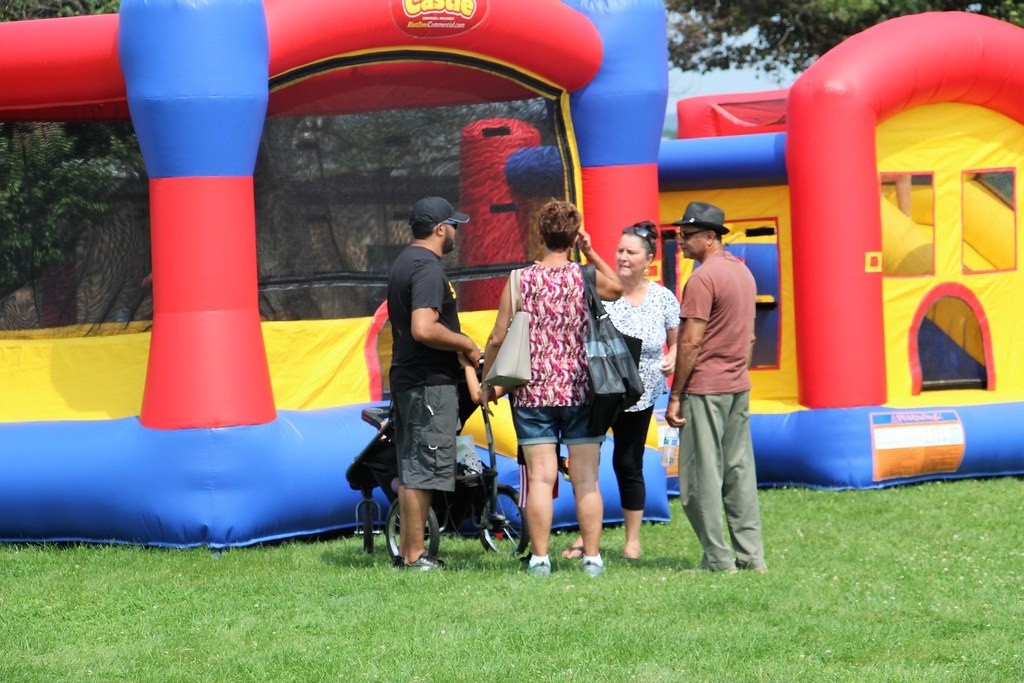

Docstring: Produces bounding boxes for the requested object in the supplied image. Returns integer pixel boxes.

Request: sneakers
[393,555,448,571]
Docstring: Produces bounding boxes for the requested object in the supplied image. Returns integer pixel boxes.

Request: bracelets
[478,382,495,390]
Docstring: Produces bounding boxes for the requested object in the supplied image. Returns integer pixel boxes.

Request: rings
[481,407,485,409]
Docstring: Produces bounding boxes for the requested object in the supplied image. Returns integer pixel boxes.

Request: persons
[664,201,769,575]
[386,197,680,578]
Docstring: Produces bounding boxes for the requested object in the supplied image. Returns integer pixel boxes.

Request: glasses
[675,230,709,239]
[442,220,458,229]
[623,226,654,252]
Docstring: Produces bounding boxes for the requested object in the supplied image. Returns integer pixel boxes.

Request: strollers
[344,353,533,567]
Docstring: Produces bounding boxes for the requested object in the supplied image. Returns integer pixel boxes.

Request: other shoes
[583,558,604,575]
[526,560,551,579]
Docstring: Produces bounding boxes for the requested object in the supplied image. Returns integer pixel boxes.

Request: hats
[409,197,470,227]
[671,202,730,234]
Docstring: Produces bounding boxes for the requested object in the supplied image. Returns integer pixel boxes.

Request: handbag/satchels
[483,269,532,388]
[579,264,645,436]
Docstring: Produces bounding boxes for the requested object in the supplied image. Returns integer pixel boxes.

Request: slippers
[563,546,585,559]
